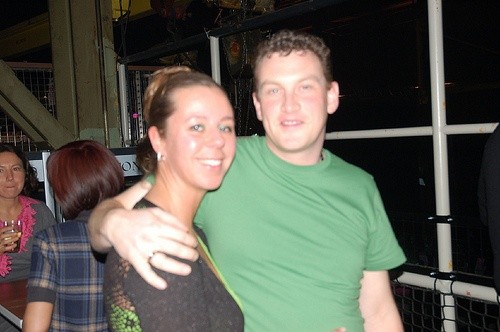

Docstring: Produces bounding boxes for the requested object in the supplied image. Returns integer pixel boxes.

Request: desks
[0,278,29,329]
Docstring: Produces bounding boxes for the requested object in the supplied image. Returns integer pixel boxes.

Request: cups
[3,219,21,253]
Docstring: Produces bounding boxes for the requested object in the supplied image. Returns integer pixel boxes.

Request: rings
[148,251,163,265]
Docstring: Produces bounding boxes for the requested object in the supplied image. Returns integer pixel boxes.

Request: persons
[22,139,126,332]
[88,32,407,332]
[478,121,500,296]
[0,141,63,332]
[103,64,245,332]
